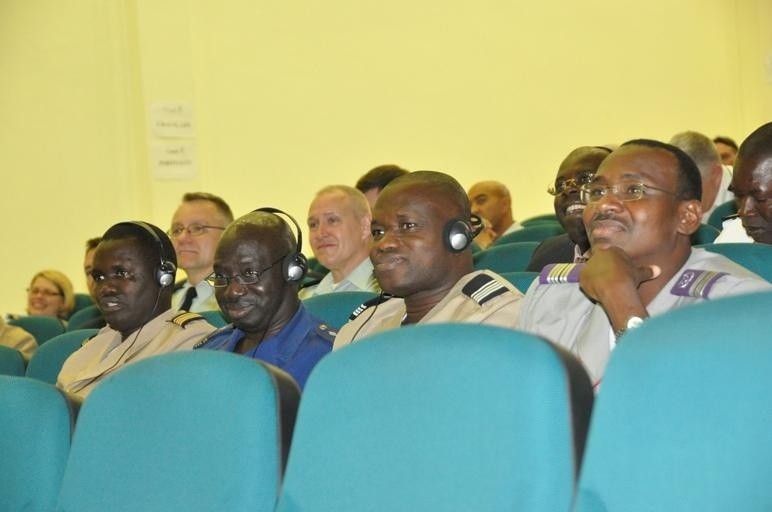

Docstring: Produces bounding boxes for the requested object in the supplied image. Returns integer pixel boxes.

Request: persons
[333,170,525,352]
[52,220,219,400]
[355,164,411,213]
[714,136,738,167]
[25,269,77,330]
[193,209,341,392]
[166,191,233,312]
[466,181,526,251]
[84,236,106,303]
[516,139,772,402]
[296,183,382,301]
[667,129,738,223]
[0,315,40,362]
[527,145,614,273]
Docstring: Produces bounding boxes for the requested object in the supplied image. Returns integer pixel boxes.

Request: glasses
[204,256,286,288]
[544,173,598,196]
[166,222,226,238]
[578,173,674,205]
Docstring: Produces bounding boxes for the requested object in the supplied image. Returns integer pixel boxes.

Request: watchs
[615,315,650,342]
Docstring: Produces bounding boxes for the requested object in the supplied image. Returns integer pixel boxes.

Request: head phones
[444,214,485,253]
[123,221,176,288]
[251,207,307,284]
